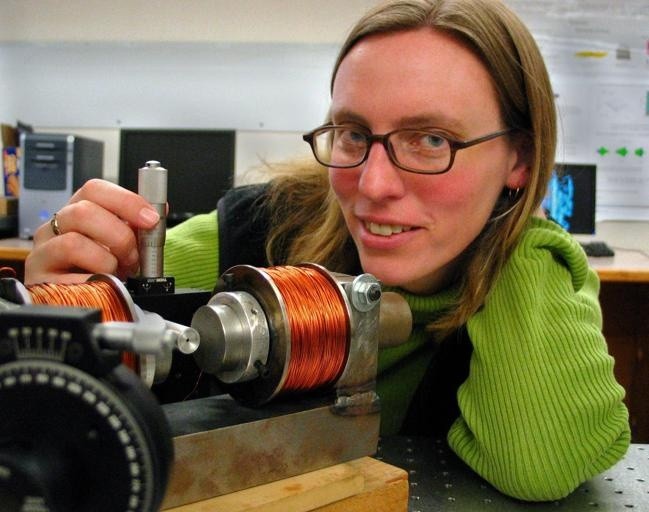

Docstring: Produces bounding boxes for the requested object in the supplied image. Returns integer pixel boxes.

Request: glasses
[299,117,518,177]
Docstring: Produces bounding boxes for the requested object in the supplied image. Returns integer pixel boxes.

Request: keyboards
[581,242,613,257]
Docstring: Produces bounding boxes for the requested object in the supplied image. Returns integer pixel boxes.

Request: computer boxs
[15,133,106,242]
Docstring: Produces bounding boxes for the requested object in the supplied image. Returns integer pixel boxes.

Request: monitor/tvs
[540,163,598,235]
[119,126,237,229]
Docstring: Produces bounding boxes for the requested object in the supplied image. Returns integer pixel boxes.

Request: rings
[50,212,61,235]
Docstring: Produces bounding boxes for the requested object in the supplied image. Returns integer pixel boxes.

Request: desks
[0,238,648,445]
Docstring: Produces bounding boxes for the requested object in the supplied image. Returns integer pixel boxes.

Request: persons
[23,0,632,505]
